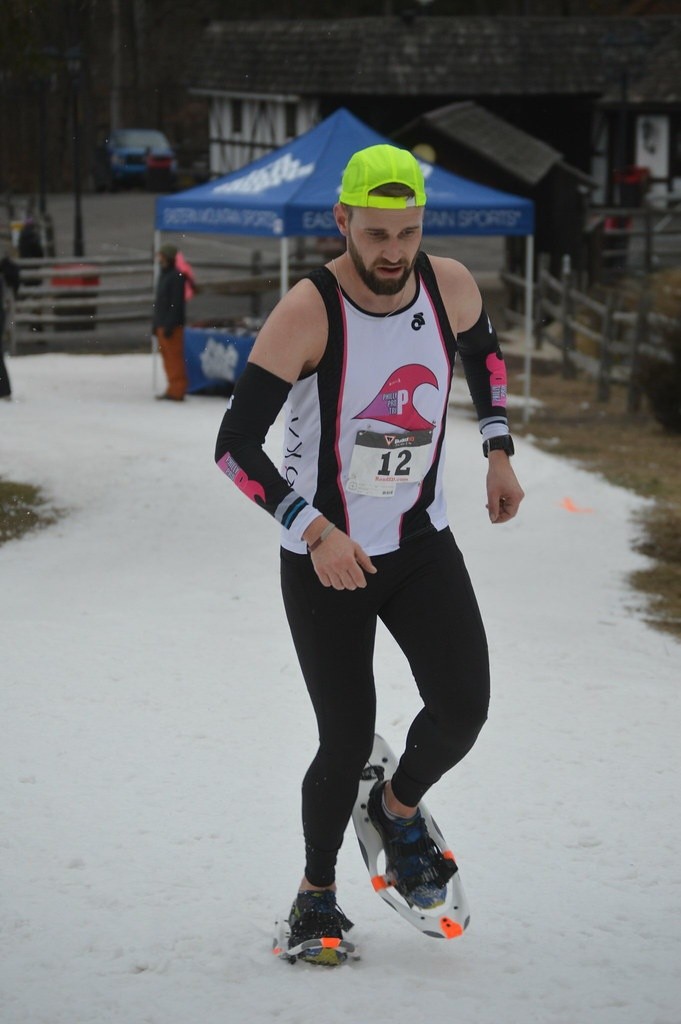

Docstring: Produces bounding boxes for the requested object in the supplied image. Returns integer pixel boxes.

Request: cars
[95,128,177,194]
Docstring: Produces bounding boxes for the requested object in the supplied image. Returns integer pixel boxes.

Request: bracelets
[308,523,334,552]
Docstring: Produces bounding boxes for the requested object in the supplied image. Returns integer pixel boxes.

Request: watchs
[483,435,516,458]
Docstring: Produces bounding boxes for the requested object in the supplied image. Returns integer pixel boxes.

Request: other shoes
[155,394,169,400]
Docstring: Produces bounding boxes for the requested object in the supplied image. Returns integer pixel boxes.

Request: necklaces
[331,259,407,321]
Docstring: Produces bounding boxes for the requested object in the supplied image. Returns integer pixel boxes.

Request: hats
[160,243,177,272]
[339,145,426,209]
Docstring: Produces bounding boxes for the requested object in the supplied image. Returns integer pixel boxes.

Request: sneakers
[288,890,354,967]
[366,780,447,909]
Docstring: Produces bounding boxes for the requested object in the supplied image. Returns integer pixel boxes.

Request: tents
[152,109,535,430]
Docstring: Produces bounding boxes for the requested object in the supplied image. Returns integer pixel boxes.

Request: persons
[0,213,47,403]
[150,245,191,402]
[214,145,526,966]
